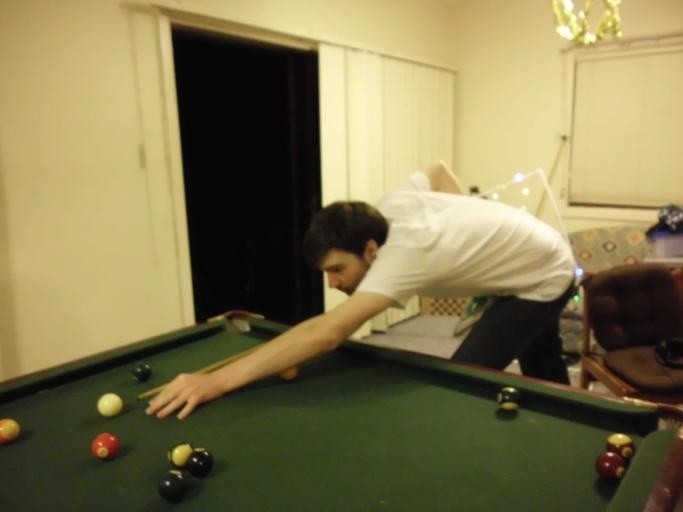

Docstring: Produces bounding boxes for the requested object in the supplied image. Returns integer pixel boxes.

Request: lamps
[549,0,624,46]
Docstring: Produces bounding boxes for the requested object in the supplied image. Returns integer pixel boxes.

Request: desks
[1,308,681,510]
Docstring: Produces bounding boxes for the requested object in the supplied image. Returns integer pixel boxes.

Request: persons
[144,159,576,419]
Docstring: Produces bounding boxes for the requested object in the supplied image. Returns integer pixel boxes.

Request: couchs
[559,224,652,357]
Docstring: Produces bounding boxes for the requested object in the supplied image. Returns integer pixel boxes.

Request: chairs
[577,262,683,400]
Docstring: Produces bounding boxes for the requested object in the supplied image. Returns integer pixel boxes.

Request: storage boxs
[419,295,473,319]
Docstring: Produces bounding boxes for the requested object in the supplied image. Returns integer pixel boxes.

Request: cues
[138,344,258,399]
[535,135,567,216]
